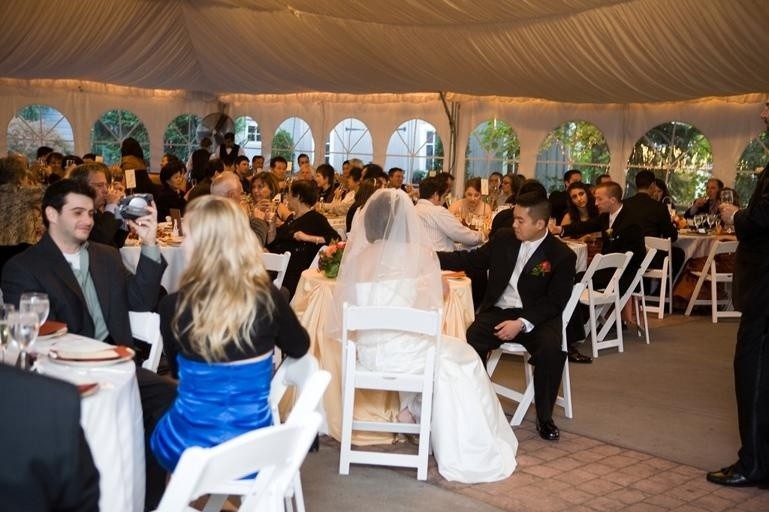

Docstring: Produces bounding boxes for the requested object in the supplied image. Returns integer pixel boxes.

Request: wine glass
[0,290,51,373]
[677,189,735,234]
[37,157,48,182]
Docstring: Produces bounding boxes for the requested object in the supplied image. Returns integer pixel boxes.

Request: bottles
[319,197,326,216]
[490,184,499,210]
[171,219,179,241]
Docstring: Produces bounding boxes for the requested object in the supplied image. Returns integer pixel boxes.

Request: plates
[160,222,183,245]
[36,321,136,396]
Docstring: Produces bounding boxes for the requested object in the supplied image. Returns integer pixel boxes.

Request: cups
[337,183,346,195]
[66,160,76,168]
[266,203,277,223]
[104,195,123,204]
[446,197,453,208]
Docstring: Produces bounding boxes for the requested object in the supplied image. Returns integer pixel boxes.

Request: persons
[685,176,724,230]
[0,134,678,512]
[706,99,769,487]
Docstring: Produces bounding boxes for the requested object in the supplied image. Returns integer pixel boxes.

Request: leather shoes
[567,347,593,364]
[706,461,769,491]
[535,412,560,441]
